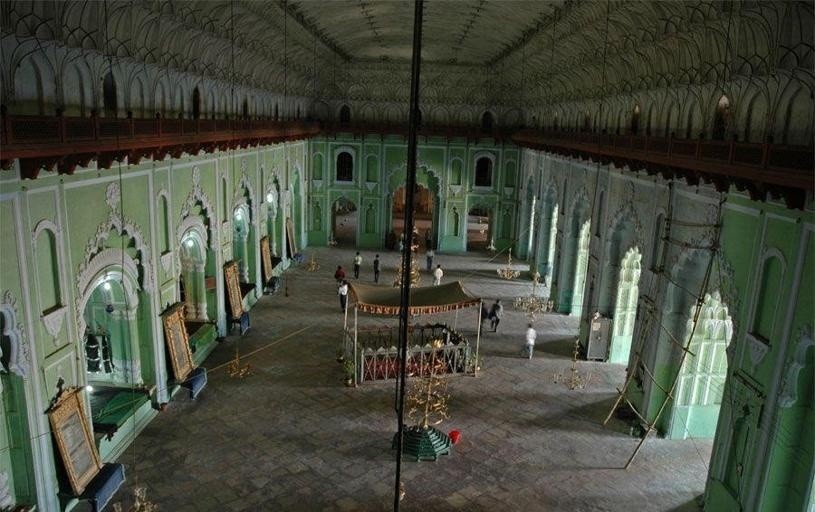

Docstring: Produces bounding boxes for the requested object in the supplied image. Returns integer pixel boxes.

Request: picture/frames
[261,236,273,282]
[287,217,297,258]
[43,386,104,496]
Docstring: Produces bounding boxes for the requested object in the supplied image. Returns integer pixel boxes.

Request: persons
[373,254,381,284]
[425,247,434,272]
[480,302,488,335]
[490,299,503,332]
[425,228,433,250]
[433,264,443,286]
[338,279,348,313]
[520,322,537,358]
[354,251,362,278]
[335,265,346,296]
[390,222,419,254]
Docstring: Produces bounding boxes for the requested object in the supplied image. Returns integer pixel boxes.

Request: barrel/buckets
[449,430,460,444]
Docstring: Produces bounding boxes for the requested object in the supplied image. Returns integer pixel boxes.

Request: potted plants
[335,342,345,363]
[343,360,355,385]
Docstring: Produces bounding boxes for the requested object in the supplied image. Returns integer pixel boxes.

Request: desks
[291,254,303,267]
[263,277,280,295]
[57,462,126,512]
